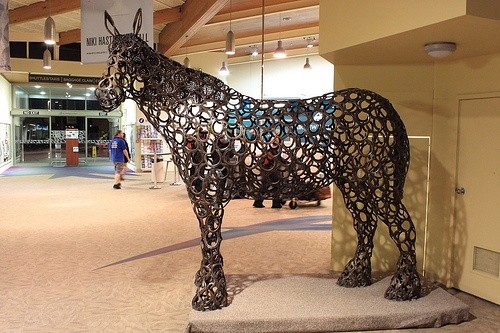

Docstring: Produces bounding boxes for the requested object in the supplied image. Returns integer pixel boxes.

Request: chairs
[166,158,181,185]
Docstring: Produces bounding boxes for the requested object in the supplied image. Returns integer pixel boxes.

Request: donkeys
[95,7,422,312]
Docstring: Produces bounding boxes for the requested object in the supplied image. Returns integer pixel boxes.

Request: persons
[210,132,234,204]
[115,133,131,180]
[108,130,130,189]
[190,130,208,192]
[253,130,286,208]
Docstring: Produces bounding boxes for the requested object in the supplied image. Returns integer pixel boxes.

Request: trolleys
[280,165,332,209]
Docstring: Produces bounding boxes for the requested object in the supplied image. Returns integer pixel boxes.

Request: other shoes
[271,204,282,208]
[253,201,265,207]
[113,183,121,189]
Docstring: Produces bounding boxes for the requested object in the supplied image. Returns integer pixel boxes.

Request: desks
[142,138,165,189]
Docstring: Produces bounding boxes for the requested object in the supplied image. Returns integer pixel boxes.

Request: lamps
[273,13,286,59]
[249,45,259,57]
[304,48,312,70]
[306,38,313,48]
[183,36,190,68]
[44,0,55,45]
[225,0,236,54]
[425,42,455,56]
[219,62,229,75]
[43,45,51,70]
